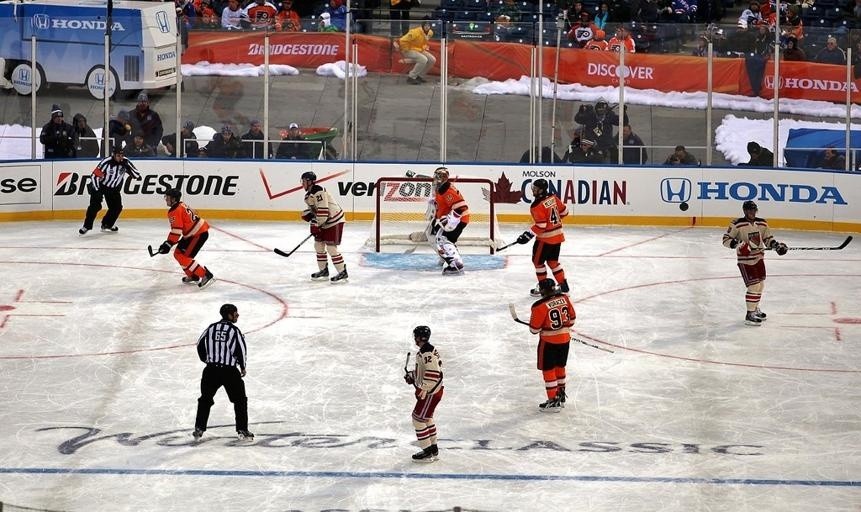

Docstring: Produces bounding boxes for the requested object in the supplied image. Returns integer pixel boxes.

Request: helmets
[743,201,757,210]
[533,279,555,297]
[302,172,316,180]
[432,168,449,186]
[220,305,239,323]
[112,146,123,154]
[166,188,179,202]
[414,326,430,337]
[534,179,548,191]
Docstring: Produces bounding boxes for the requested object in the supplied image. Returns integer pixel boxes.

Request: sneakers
[444,265,463,272]
[79,226,91,233]
[531,288,540,294]
[746,311,761,322]
[183,277,199,282]
[417,76,426,82]
[312,268,329,277]
[412,447,432,459]
[101,221,118,231]
[755,309,766,318]
[431,445,438,453]
[193,429,203,437]
[238,430,254,437]
[407,77,419,84]
[198,273,213,287]
[558,280,569,293]
[539,400,559,408]
[331,271,348,281]
[558,392,566,402]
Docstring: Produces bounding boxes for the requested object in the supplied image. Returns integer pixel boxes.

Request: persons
[156,188,217,289]
[814,145,851,172]
[528,278,577,412]
[36,90,336,159]
[178,0,861,94]
[404,325,445,460]
[517,178,571,295]
[664,145,699,166]
[739,141,775,167]
[189,304,255,438]
[722,200,788,326]
[429,166,471,275]
[299,171,350,283]
[78,147,143,237]
[563,97,650,165]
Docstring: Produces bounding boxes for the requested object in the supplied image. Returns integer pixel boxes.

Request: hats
[183,121,194,130]
[580,138,594,146]
[290,123,298,129]
[51,104,63,117]
[119,111,130,120]
[138,93,148,102]
[222,126,231,134]
[134,129,143,137]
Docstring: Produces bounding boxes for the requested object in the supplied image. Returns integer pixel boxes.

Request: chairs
[214,1,368,30]
[797,0,861,64]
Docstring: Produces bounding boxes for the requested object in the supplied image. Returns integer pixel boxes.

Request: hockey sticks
[147,241,179,257]
[404,220,430,255]
[404,352,417,388]
[509,303,614,353]
[274,234,313,257]
[489,238,518,251]
[751,236,853,250]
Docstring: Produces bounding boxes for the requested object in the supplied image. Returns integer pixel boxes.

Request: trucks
[1,0,183,102]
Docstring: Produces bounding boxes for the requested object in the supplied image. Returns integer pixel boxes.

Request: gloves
[415,390,426,400]
[736,240,751,256]
[770,240,788,255]
[518,232,533,244]
[405,371,414,384]
[311,223,321,237]
[160,242,171,253]
[302,210,314,222]
